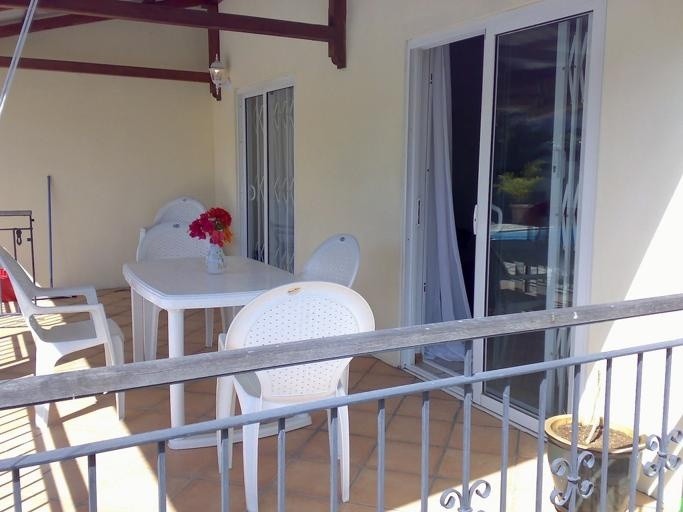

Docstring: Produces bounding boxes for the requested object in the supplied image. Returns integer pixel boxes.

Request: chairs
[147,198,209,227]
[135,220,215,361]
[211,279,378,511]
[0,245,127,432]
[295,233,362,291]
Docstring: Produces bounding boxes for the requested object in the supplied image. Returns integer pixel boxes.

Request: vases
[542,409,647,512]
[204,242,228,275]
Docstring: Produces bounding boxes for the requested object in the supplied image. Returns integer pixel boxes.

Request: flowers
[187,206,235,248]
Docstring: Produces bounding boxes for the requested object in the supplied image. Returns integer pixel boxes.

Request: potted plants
[494,171,549,226]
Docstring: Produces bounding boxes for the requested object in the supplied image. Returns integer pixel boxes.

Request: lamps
[207,52,232,95]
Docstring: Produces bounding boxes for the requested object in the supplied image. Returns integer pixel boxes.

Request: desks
[119,254,315,453]
